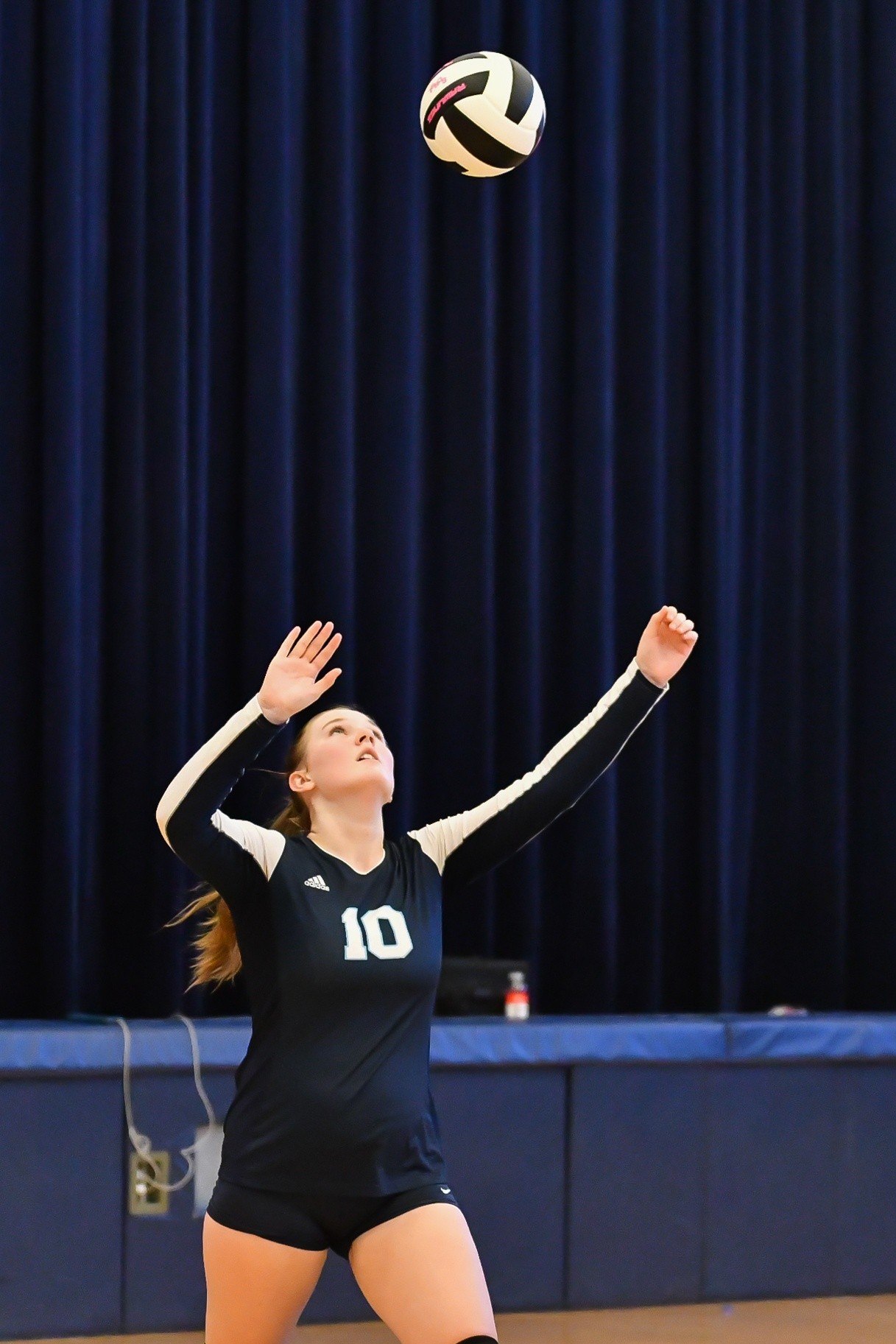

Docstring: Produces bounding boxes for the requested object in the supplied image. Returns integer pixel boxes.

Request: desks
[1,1011,896,1341]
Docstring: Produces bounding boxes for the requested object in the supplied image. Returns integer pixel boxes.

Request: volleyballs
[420,51,547,178]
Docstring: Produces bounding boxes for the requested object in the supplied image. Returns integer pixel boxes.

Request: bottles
[504,971,530,1021]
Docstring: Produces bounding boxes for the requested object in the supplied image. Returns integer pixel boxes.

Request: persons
[153,605,697,1344]
